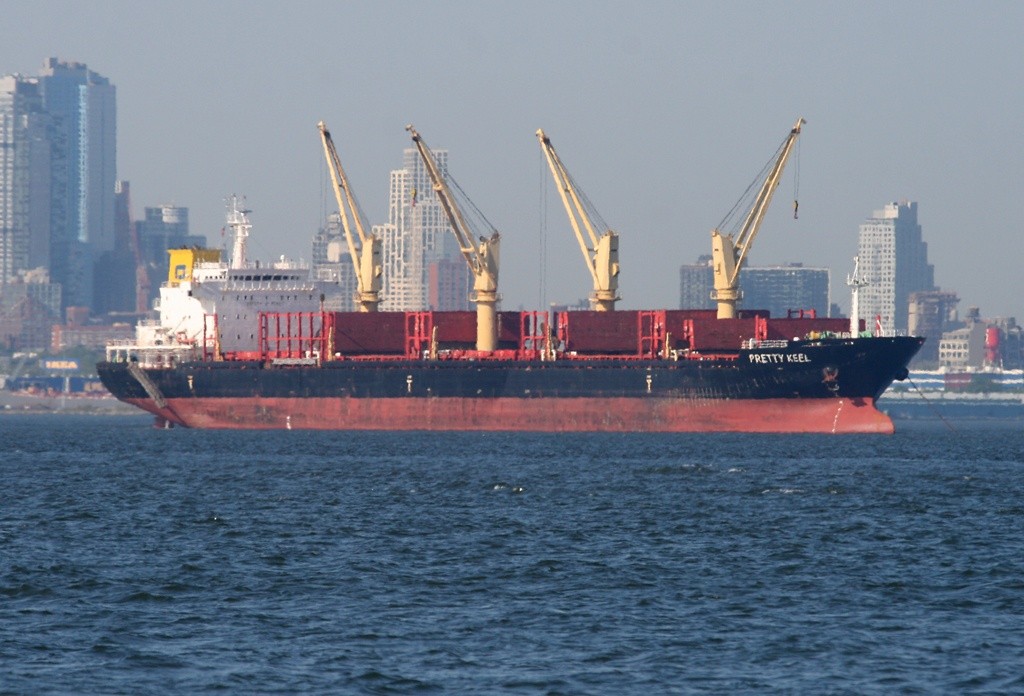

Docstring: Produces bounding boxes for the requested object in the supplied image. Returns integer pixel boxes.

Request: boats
[95,117,931,437]
